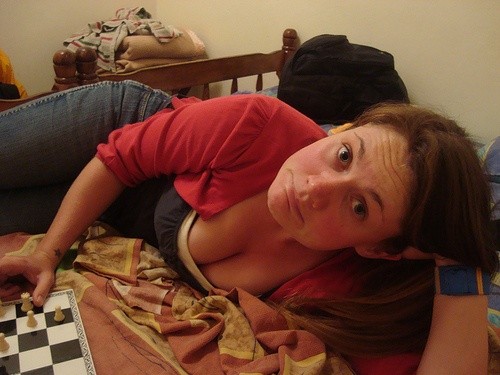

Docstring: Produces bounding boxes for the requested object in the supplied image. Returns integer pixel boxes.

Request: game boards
[0,290,94,375]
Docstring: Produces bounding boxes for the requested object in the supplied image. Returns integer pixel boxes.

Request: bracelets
[434,264,490,295]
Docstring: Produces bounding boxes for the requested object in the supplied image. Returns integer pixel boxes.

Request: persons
[0,79,494,375]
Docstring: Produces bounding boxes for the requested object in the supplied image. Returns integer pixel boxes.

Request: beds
[1,29,500,375]
